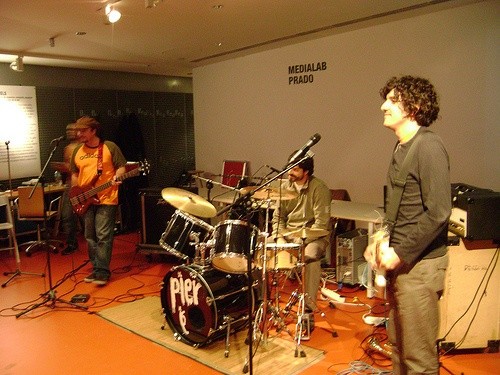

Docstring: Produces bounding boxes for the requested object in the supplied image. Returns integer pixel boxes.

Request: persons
[70,117,126,285]
[257,150,332,340]
[363,75,451,375]
[53,123,85,253]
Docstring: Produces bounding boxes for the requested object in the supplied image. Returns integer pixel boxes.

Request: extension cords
[320,288,345,303]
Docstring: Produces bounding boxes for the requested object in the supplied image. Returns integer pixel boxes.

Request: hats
[75,116,100,129]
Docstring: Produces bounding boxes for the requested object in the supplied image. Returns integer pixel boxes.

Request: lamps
[103,4,121,22]
[9,54,24,72]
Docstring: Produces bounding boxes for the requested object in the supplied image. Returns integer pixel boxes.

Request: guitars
[368,231,390,300]
[69,158,152,215]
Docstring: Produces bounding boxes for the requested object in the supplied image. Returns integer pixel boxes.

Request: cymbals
[238,186,300,200]
[161,187,218,218]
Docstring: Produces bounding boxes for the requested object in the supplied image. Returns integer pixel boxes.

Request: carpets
[97,296,324,375]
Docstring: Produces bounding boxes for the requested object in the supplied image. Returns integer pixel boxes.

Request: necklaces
[85,143,98,148]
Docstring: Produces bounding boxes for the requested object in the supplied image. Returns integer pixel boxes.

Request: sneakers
[93,277,107,284]
[84,273,97,281]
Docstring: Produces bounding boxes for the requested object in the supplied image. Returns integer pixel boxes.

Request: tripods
[216,145,338,374]
[2,140,88,317]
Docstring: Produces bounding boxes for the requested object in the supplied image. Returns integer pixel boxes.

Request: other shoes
[63,242,77,253]
[301,311,314,332]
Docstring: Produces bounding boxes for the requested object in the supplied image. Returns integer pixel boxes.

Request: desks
[0,183,65,199]
[200,186,385,297]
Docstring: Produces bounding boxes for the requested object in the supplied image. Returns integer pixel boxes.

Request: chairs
[14,186,64,256]
[0,192,21,262]
[222,160,245,187]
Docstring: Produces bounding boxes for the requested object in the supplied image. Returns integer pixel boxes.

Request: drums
[160,259,258,346]
[158,209,215,260]
[255,243,302,271]
[211,219,261,274]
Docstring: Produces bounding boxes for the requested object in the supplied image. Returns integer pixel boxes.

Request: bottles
[54,169,63,185]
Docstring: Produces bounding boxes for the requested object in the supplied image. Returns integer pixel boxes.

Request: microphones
[50,136,65,145]
[265,165,280,173]
[283,133,322,169]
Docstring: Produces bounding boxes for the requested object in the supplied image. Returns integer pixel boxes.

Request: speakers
[138,186,203,252]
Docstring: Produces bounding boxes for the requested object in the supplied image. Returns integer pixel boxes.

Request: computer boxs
[335,227,368,286]
